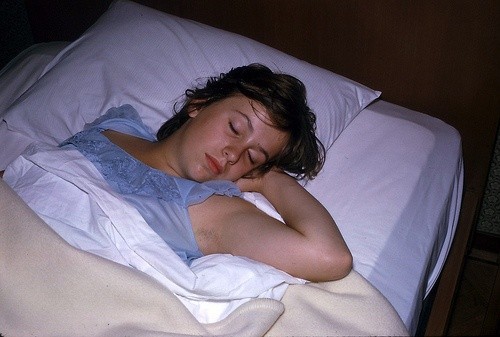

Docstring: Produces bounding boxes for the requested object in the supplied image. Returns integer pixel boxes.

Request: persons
[56,61,354,283]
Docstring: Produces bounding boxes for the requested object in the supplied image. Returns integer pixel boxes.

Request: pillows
[2,0,382,194]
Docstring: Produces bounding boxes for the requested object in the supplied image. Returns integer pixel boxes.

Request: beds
[0,0,500,337]
